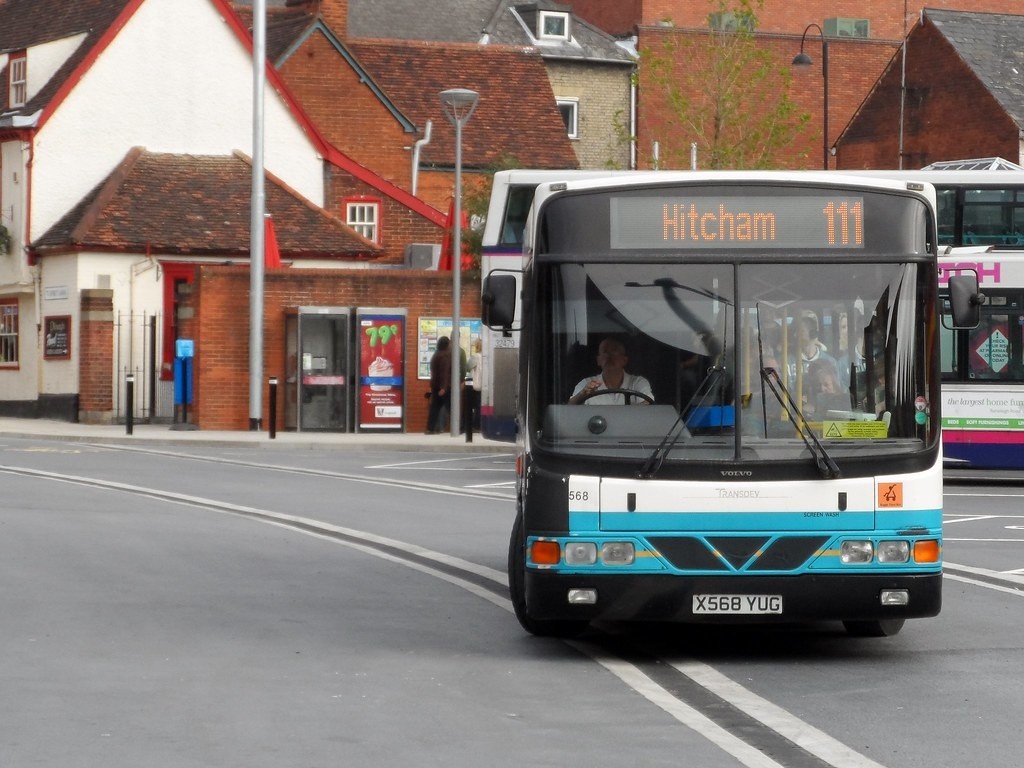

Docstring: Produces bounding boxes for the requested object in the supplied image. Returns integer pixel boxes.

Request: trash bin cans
[424,392,451,432]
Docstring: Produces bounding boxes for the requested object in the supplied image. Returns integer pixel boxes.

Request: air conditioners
[403,243,442,271]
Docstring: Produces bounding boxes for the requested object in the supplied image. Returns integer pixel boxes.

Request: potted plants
[656,17,675,28]
[705,0,764,31]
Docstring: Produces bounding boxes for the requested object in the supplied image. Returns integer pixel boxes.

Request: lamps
[613,31,640,59]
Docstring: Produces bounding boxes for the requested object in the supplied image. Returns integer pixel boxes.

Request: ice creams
[367,356,395,392]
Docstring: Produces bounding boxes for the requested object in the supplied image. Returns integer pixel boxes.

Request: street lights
[436,86,481,438]
[791,22,830,171]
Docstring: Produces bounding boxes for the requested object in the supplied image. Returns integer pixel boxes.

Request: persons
[426,331,482,433]
[570,338,654,405]
[717,307,884,416]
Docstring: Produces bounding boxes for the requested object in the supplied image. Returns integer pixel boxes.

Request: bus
[477,166,1024,489]
[482,166,988,643]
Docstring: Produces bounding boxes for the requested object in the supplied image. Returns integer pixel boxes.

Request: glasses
[599,349,626,358]
